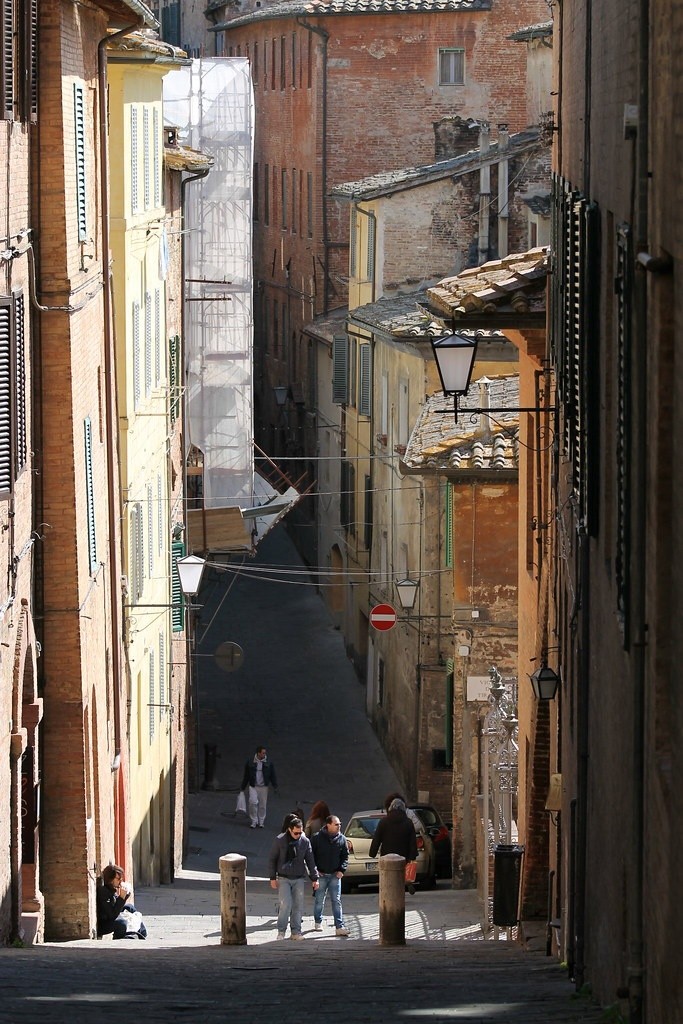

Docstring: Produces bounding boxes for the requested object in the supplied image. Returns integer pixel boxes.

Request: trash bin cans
[493,845,522,927]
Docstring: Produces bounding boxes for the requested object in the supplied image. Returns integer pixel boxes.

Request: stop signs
[371,605,397,632]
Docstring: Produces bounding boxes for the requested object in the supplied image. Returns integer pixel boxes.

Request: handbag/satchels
[235,791,246,812]
[405,860,418,884]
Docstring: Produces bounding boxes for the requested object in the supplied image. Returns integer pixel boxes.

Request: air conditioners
[164,126,178,148]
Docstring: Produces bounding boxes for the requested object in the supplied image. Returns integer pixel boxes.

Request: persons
[304,800,331,896]
[369,799,417,864]
[96,864,148,940]
[282,815,306,922]
[239,745,279,828]
[269,818,319,941]
[309,815,351,936]
[385,793,422,895]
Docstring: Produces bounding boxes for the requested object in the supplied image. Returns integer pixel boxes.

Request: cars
[339,803,452,894]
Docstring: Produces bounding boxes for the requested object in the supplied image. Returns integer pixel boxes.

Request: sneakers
[315,923,323,931]
[250,819,258,828]
[259,818,265,827]
[336,928,350,935]
[277,932,284,940]
[291,935,305,940]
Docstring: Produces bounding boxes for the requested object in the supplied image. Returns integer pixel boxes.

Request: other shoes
[408,885,415,895]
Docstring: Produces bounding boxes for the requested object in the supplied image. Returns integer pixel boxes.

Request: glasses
[292,831,302,835]
[115,877,121,880]
[335,823,342,826]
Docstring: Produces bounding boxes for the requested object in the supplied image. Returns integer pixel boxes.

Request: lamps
[175,549,207,597]
[429,315,479,425]
[525,646,560,702]
[394,569,420,617]
